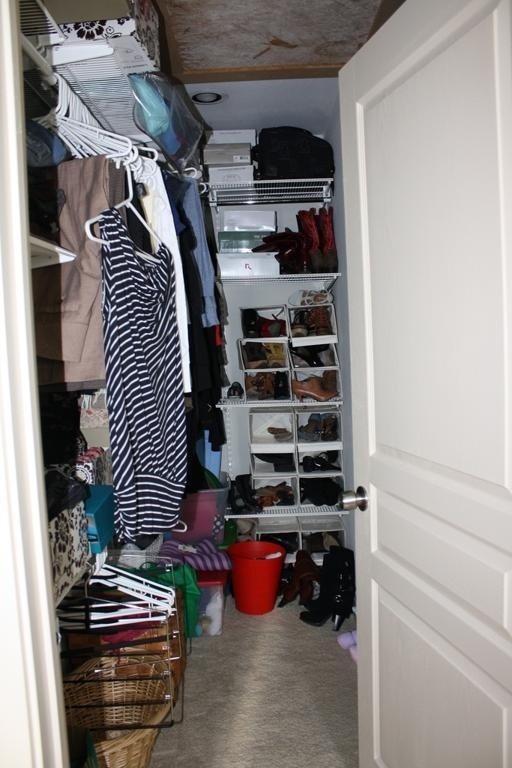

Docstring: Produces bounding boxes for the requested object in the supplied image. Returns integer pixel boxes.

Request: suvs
[227,540,287,616]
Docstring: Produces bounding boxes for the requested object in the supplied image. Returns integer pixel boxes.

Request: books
[203,129,256,182]
[215,210,280,279]
[43,447,231,635]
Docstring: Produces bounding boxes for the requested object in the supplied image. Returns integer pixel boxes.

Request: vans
[292,379,337,402]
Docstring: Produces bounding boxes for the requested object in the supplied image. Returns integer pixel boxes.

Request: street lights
[251,127,335,201]
[110,561,201,638]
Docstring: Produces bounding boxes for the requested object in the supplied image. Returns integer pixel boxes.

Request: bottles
[159,538,232,572]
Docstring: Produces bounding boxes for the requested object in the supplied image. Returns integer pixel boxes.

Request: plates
[216,177,350,565]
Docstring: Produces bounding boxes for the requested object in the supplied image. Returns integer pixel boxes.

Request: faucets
[288,289,334,305]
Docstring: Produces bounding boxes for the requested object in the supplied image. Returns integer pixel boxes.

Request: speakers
[292,307,333,336]
[274,373,290,399]
[297,414,337,441]
[253,207,337,274]
[246,376,257,395]
[278,545,356,632]
[322,370,337,390]
[257,372,274,400]
[229,475,263,514]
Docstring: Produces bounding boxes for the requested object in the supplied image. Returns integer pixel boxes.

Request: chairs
[314,452,329,467]
[303,456,313,472]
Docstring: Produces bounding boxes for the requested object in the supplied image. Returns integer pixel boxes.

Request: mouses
[85,158,172,268]
[30,72,211,196]
[171,519,187,533]
